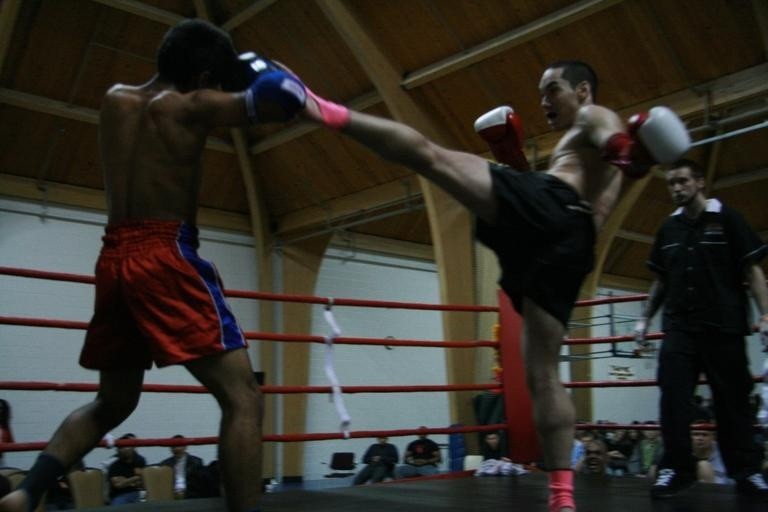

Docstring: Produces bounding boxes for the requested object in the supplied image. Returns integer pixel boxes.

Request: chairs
[734,471,767,509]
[649,467,699,497]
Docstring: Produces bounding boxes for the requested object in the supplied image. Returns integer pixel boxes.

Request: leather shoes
[758,314,768,353]
[633,315,650,348]
[627,104,693,173]
[472,105,530,171]
[241,70,308,125]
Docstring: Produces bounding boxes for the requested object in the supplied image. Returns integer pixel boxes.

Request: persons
[632,156,766,499]
[351,433,397,486]
[566,392,767,485]
[480,428,502,459]
[392,423,443,479]
[0,398,15,443]
[236,50,693,512]
[102,432,221,503]
[1,17,310,512]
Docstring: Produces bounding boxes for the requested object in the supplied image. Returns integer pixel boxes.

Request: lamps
[0,461,178,509]
[314,450,359,487]
